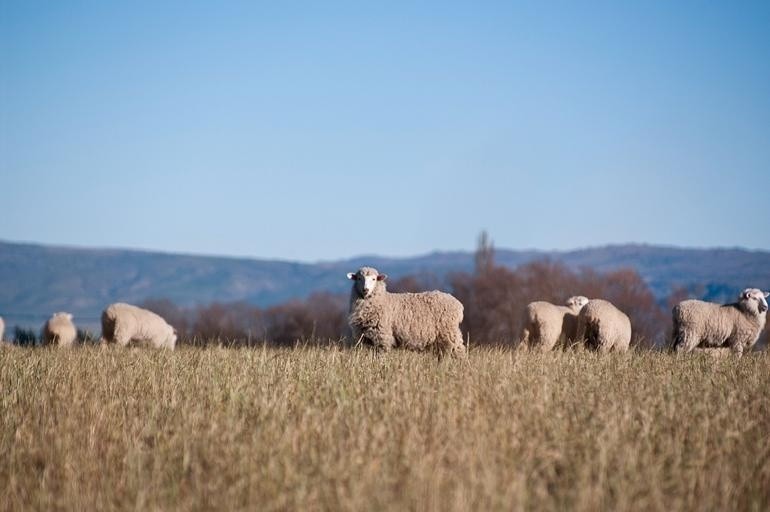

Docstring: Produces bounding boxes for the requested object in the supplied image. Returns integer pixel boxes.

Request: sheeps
[575,298,632,360]
[667,288,770,359]
[518,295,589,351]
[346,266,467,371]
[99,303,178,353]
[44,311,77,350]
